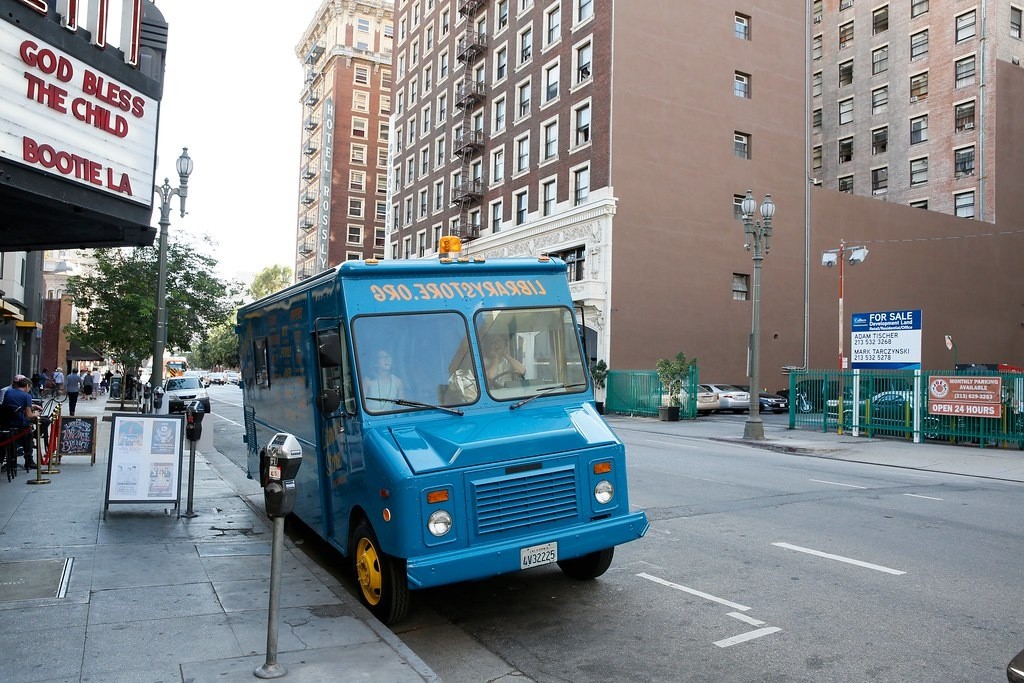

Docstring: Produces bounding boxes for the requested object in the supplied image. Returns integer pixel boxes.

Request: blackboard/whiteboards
[57,416,98,455]
[110,378,123,399]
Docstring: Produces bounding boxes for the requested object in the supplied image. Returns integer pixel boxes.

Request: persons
[360,347,406,413]
[37,368,53,397]
[78,368,100,401]
[482,331,526,390]
[0,374,50,474]
[100,369,123,395]
[117,465,138,482]
[64,368,84,416]
[53,367,64,397]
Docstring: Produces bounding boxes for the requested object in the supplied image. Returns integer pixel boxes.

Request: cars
[734,385,788,414]
[701,385,751,414]
[839,394,993,439]
[635,383,721,415]
[864,378,913,397]
[187,371,243,388]
[776,381,840,414]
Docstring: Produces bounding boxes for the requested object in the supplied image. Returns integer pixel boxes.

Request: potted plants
[589,359,608,415]
[655,352,696,421]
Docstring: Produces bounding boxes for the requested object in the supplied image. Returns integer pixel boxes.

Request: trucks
[237,235,645,628]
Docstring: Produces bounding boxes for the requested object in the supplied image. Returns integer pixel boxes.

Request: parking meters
[141,383,152,414]
[153,387,167,415]
[255,432,305,678]
[184,402,208,521]
[132,378,138,403]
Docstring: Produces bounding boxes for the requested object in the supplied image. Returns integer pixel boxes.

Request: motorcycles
[772,387,812,413]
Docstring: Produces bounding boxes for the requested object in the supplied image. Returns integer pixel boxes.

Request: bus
[162,358,188,378]
[163,377,211,413]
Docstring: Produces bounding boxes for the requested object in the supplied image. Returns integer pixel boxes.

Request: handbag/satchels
[442,368,477,404]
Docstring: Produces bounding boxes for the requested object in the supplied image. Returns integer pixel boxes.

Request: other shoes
[93,398,96,400]
[70,412,75,416]
[90,397,92,400]
[24,461,37,469]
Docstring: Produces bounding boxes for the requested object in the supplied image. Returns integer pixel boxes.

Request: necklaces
[377,377,392,410]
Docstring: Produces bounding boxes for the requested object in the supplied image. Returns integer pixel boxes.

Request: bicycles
[40,380,68,404]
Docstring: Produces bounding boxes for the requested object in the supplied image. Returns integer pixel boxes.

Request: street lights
[742,188,777,441]
[820,246,873,391]
[156,147,193,404]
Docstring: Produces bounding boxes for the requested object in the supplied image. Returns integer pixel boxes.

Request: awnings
[66,342,104,362]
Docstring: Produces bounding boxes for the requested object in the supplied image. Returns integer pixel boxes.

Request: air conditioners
[911,96,918,103]
[843,0,853,9]
[816,16,821,21]
[965,123,973,129]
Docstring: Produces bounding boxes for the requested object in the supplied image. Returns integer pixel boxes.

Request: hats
[55,366,63,371]
[14,375,26,382]
